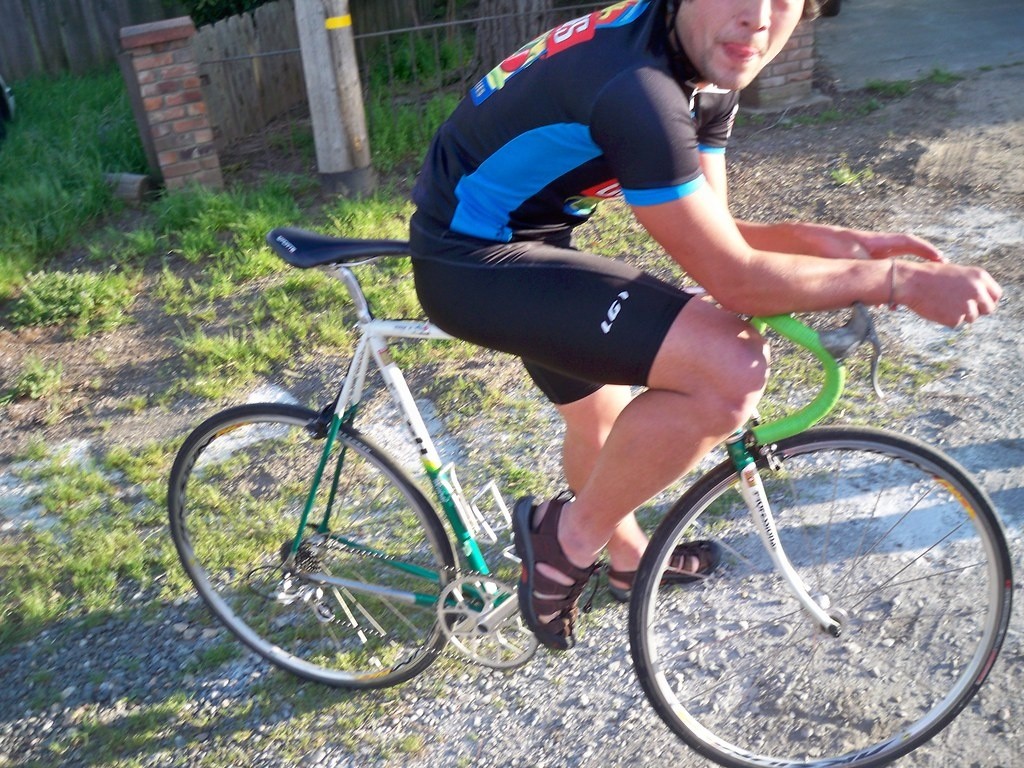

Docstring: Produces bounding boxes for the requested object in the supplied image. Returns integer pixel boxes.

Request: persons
[409,0,1005,649]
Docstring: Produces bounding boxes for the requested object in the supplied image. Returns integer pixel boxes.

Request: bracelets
[890,258,896,308]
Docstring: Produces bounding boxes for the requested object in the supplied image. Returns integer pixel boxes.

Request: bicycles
[164,220,1015,768]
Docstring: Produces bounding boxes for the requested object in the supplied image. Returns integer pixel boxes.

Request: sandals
[511,488,604,652]
[604,540,722,599]
